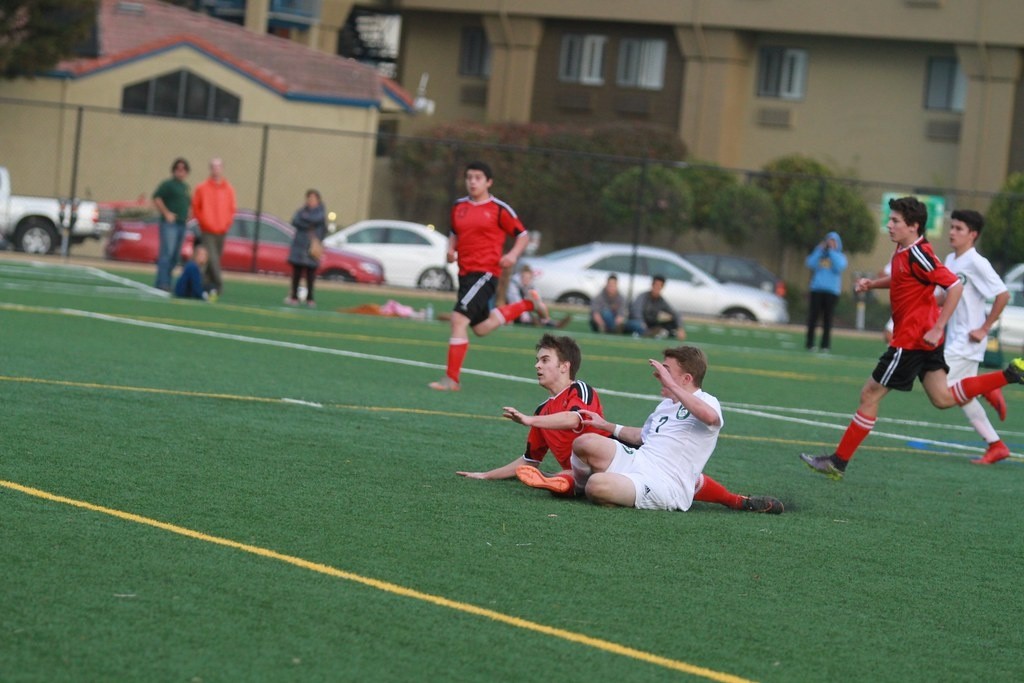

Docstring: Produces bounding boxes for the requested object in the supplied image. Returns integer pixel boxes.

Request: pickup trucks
[0,167,101,254]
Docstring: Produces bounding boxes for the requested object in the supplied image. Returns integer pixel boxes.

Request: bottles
[419,307,426,321]
[426,301,434,321]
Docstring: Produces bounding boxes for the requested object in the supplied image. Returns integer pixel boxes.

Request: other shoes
[284,295,298,303]
[306,300,315,308]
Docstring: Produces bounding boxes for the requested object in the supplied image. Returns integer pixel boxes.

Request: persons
[505,267,572,330]
[174,245,217,300]
[804,232,849,353]
[589,274,647,337]
[456,333,786,513]
[800,194,1024,479]
[569,346,726,512]
[283,188,327,308]
[934,210,1011,464]
[878,260,895,343]
[151,158,193,293]
[628,275,688,340]
[192,157,237,293]
[427,161,549,393]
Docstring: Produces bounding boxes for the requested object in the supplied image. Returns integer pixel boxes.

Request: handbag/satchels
[308,236,324,263]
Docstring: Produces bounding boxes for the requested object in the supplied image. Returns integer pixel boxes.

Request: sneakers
[970,446,1010,465]
[800,453,845,481]
[738,494,784,514]
[1005,358,1024,385]
[515,466,569,494]
[525,289,549,319]
[982,387,1006,421]
[427,376,459,390]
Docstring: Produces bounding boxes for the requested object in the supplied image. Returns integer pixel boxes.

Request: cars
[105,208,385,285]
[510,242,790,325]
[321,220,459,292]
[883,264,1024,349]
[683,251,789,296]
